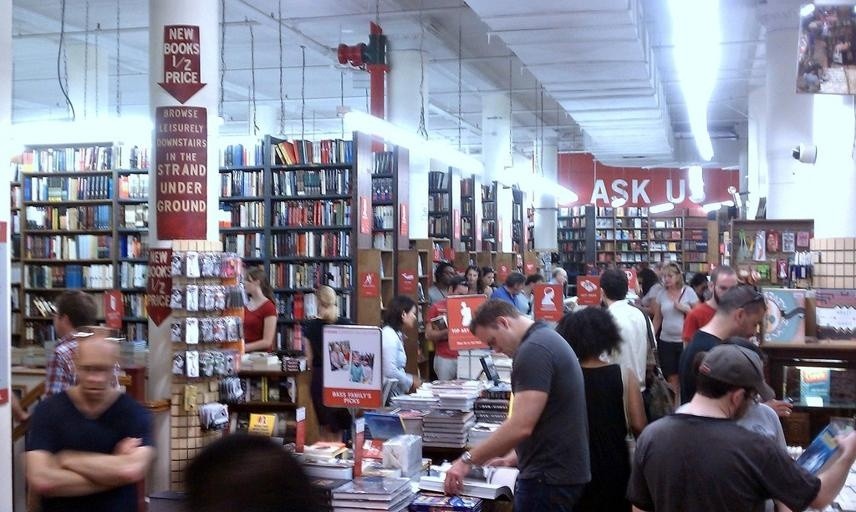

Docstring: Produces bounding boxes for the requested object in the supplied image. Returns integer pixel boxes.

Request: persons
[349,352,373,383]
[328,341,350,371]
[456,265,492,298]
[444,299,591,512]
[550,267,575,312]
[688,273,709,300]
[680,265,738,348]
[423,264,454,299]
[514,275,544,314]
[491,273,526,304]
[42,288,119,404]
[25,336,156,512]
[553,308,647,511]
[302,285,352,441]
[425,277,468,380]
[652,264,699,382]
[631,269,660,315]
[180,436,329,511]
[482,266,498,297]
[243,265,278,355]
[627,343,856,512]
[382,298,421,401]
[681,283,766,405]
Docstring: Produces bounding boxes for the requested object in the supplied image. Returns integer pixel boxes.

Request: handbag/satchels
[635,306,677,423]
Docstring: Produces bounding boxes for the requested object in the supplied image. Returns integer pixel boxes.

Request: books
[220,140,267,259]
[482,185,495,252]
[270,139,355,164]
[527,207,533,252]
[272,170,350,194]
[22,144,150,287]
[814,289,856,341]
[557,205,733,276]
[295,379,519,512]
[273,231,351,257]
[272,200,351,224]
[429,172,449,238]
[270,261,353,287]
[460,178,473,251]
[794,419,853,476]
[373,151,394,252]
[121,292,147,340]
[34,292,58,318]
[513,190,523,253]
[760,287,806,343]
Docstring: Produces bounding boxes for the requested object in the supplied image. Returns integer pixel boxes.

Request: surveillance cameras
[791,145,815,163]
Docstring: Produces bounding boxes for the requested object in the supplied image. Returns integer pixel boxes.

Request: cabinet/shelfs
[596,206,616,266]
[21,141,112,334]
[428,167,462,264]
[512,190,527,271]
[405,234,433,381]
[753,287,856,441]
[685,216,710,275]
[238,363,347,450]
[557,207,596,267]
[219,138,267,293]
[113,141,150,340]
[481,181,504,266]
[617,208,650,266]
[369,149,410,309]
[461,173,481,268]
[527,207,536,250]
[266,135,382,328]
[649,212,684,272]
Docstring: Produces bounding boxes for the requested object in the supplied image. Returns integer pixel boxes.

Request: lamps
[727,186,742,208]
[504,167,584,203]
[647,203,676,211]
[685,164,706,201]
[668,1,721,162]
[699,202,735,211]
[344,110,486,174]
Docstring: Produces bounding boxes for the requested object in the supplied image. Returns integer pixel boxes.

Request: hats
[697,343,776,402]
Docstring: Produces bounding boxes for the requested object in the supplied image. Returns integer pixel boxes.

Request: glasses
[740,294,764,310]
[670,262,681,273]
[746,393,760,407]
[460,280,470,286]
